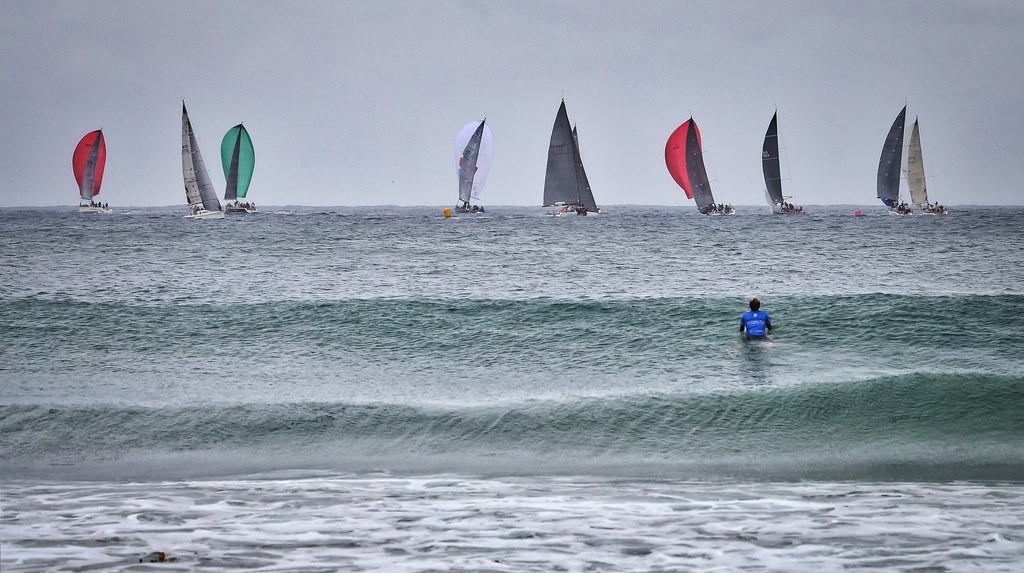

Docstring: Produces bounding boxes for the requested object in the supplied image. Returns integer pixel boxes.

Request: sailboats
[901,115,948,215]
[664,114,736,216]
[542,122,581,212]
[454,117,494,216]
[541,98,599,218]
[220,121,258,214]
[71,128,113,215]
[761,108,805,215]
[181,99,225,219]
[877,104,913,217]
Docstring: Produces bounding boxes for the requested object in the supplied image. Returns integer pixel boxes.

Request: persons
[739,298,773,339]
[225,200,257,211]
[705,203,735,214]
[780,201,803,212]
[562,203,589,216]
[461,201,484,212]
[928,201,944,212]
[91,200,110,209]
[898,202,911,214]
[189,205,205,214]
